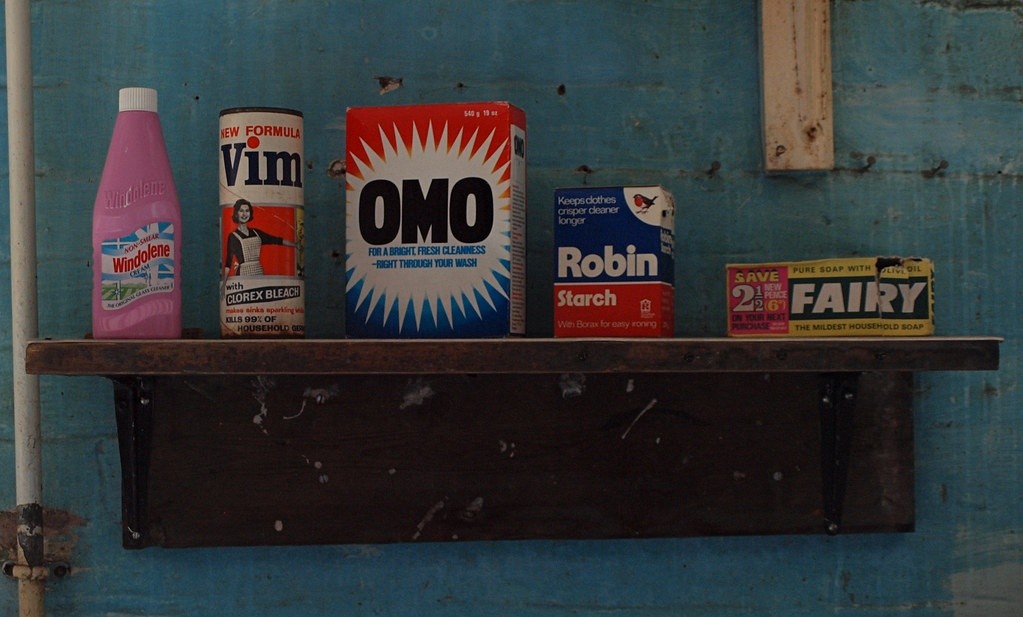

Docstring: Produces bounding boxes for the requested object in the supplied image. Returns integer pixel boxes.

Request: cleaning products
[217,106,307,339]
[91,85,184,340]
[343,100,528,341]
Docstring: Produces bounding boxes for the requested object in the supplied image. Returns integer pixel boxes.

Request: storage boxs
[726,259,936,336]
[555,185,676,338]
[345,102,527,340]
